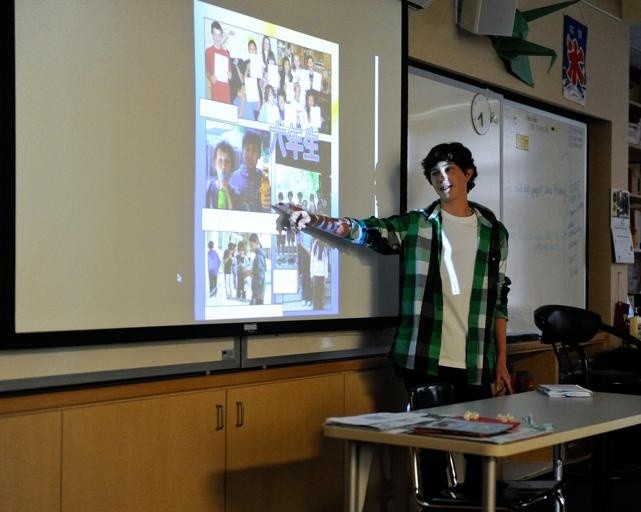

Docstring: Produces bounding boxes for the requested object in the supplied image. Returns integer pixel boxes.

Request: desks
[322,389,640,512]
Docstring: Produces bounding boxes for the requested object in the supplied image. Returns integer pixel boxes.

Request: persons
[288,143,511,512]
[205,22,331,311]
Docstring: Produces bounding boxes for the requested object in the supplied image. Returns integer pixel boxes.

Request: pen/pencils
[275,203,311,227]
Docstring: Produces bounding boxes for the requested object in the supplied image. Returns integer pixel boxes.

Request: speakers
[459,0,517,37]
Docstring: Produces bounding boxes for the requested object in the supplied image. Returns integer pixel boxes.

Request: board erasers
[506,334,539,343]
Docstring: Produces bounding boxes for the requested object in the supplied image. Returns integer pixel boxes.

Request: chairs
[406,385,568,512]
[534,304,641,479]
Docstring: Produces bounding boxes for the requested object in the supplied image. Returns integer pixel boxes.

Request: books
[325,412,555,445]
[535,384,594,397]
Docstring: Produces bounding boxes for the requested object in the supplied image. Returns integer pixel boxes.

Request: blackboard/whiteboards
[406,65,502,226]
[502,99,587,333]
